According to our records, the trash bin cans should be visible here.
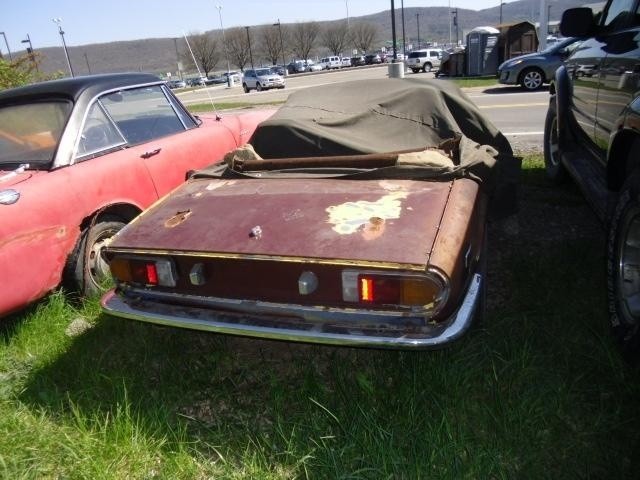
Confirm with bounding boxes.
[285,69,288,76]
[227,76,234,87]
